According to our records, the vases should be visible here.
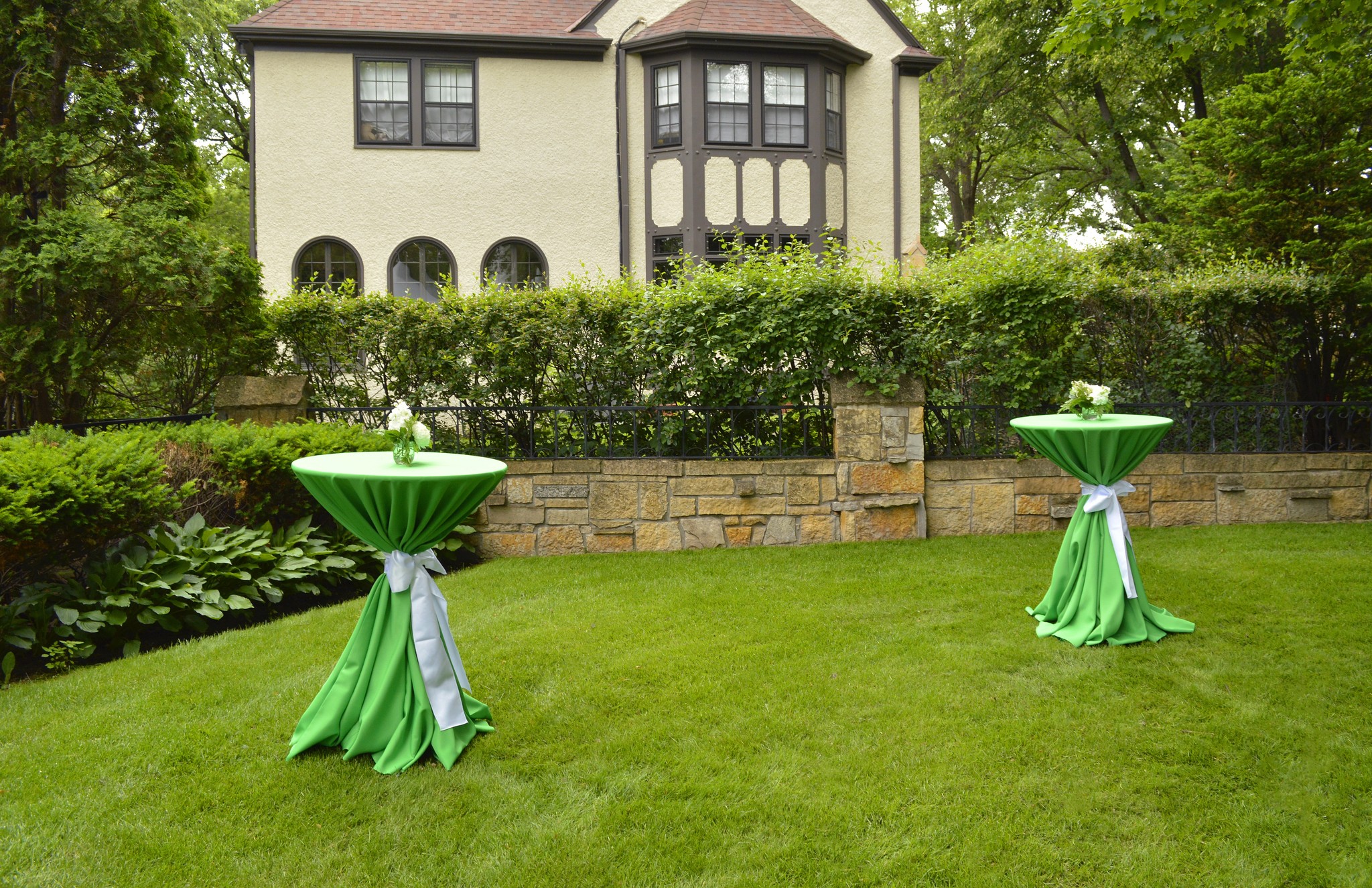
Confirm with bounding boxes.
[393,441,414,466]
[1082,406,1098,420]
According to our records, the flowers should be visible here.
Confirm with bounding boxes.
[384,400,433,451]
[1057,380,1114,419]
